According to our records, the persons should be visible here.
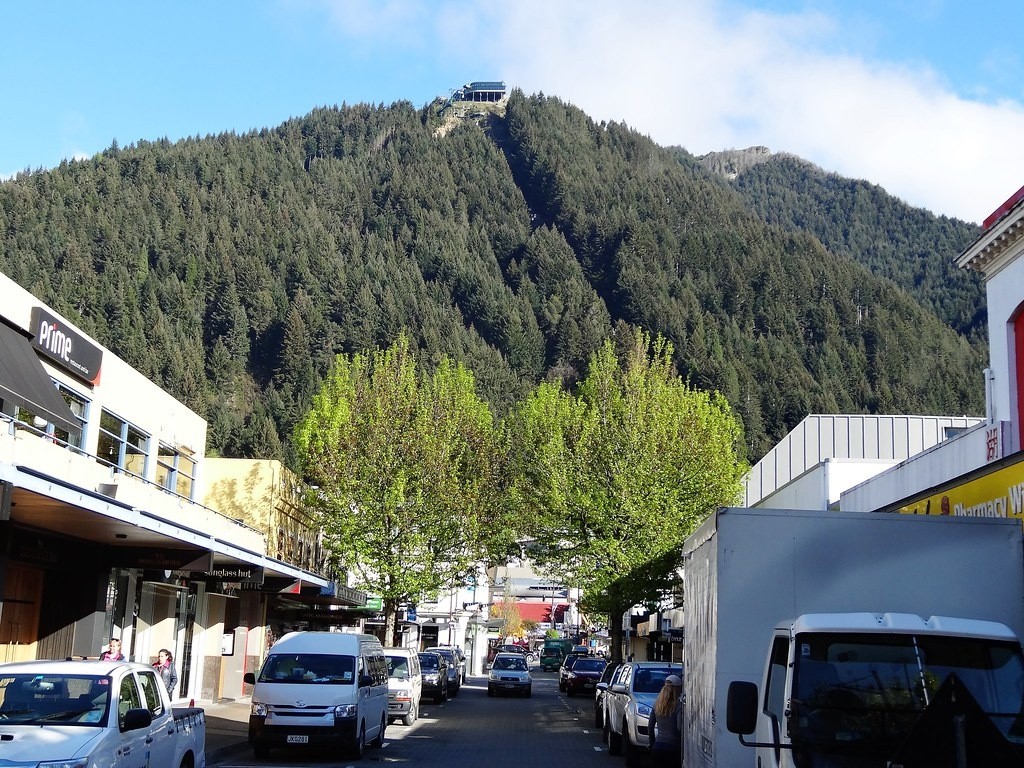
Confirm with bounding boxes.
[646,676,681,768]
[152,649,178,702]
[511,659,527,670]
[96,639,126,685]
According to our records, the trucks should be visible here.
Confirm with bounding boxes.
[540,639,572,672]
[681,505,1024,768]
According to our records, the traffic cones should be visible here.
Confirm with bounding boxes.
[189,699,194,708]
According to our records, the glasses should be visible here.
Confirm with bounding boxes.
[111,638,119,642]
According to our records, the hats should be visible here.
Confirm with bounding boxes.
[665,676,682,686]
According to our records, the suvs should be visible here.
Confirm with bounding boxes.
[486,653,533,697]
[565,657,609,697]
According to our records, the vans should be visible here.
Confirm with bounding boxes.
[244,632,389,764]
[383,647,422,726]
[571,645,588,657]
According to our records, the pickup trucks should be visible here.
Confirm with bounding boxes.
[0,660,206,768]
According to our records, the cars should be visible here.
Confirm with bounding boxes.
[559,654,589,692]
[418,652,448,704]
[423,647,463,697]
[497,644,536,662]
[607,661,684,760]
[594,663,621,729]
[596,664,624,744]
